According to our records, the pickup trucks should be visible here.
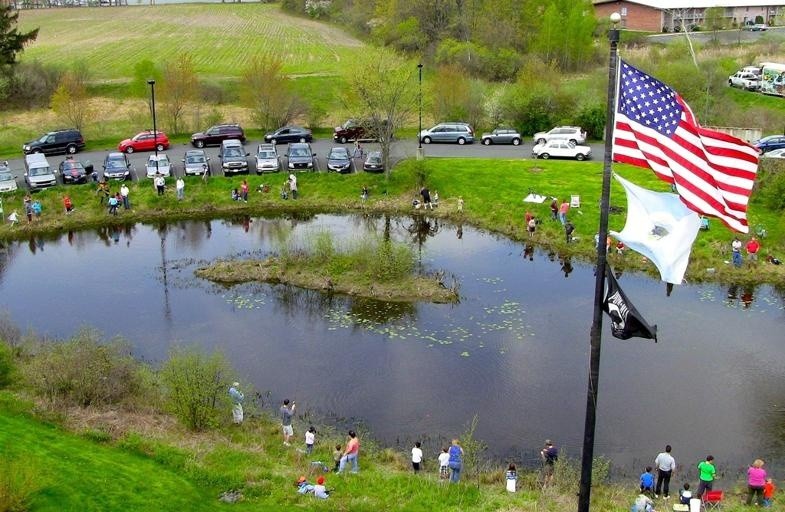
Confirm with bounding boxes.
[728,70,762,92]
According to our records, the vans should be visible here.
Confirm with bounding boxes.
[332,118,393,143]
[741,66,762,86]
[415,121,473,146]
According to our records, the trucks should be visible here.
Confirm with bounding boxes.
[758,62,785,97]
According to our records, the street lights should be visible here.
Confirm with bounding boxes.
[415,61,425,161]
[146,79,162,189]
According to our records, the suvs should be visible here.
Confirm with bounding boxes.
[23,152,58,192]
[102,152,131,182]
[325,147,353,173]
[254,141,281,174]
[748,23,767,31]
[22,126,86,156]
[0,160,18,196]
[283,143,317,172]
[191,123,246,148]
[529,122,587,145]
[218,138,249,175]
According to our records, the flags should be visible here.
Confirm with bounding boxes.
[611,171,703,286]
[599,261,660,343]
[611,55,762,233]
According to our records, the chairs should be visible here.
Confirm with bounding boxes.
[571,195,579,207]
[701,490,722,512]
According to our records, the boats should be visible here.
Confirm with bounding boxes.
[12,0,127,7]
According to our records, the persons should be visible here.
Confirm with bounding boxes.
[523,197,576,244]
[412,437,465,484]
[155,161,209,201]
[748,236,760,271]
[413,186,438,213]
[595,232,625,257]
[94,180,129,215]
[457,194,466,213]
[352,139,369,159]
[732,238,742,268]
[7,191,75,228]
[630,441,776,510]
[361,187,368,200]
[230,171,301,203]
[505,462,519,495]
[540,439,558,491]
[226,382,359,504]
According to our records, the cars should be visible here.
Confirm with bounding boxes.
[363,150,384,172]
[480,126,522,145]
[750,134,785,154]
[530,138,593,161]
[118,128,169,153]
[700,216,709,230]
[263,124,313,144]
[143,154,173,178]
[762,148,785,158]
[180,149,210,176]
[57,159,86,185]
